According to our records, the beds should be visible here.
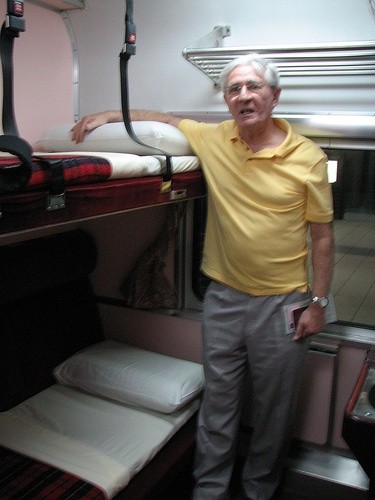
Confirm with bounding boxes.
[0,135,205,500]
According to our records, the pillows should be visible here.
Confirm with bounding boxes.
[52,340,205,414]
[34,121,194,156]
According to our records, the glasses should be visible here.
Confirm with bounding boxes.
[223,80,266,95]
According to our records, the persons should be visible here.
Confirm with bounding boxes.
[70,55,335,500]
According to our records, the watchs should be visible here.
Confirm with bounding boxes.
[311,297,329,308]
